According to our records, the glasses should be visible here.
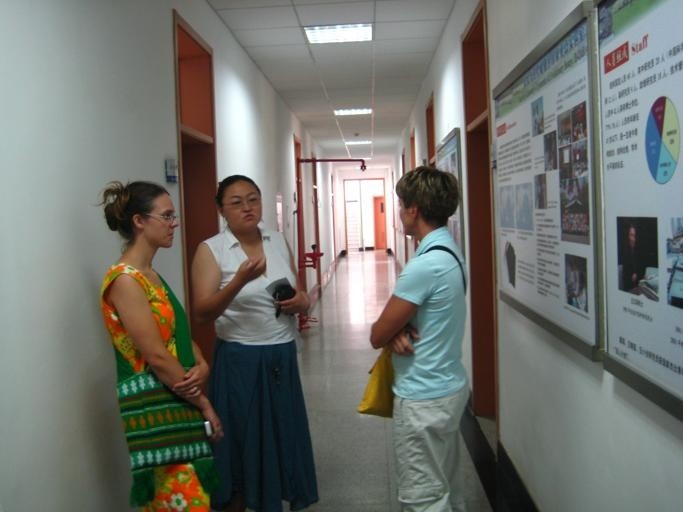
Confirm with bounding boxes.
[143,213,179,225]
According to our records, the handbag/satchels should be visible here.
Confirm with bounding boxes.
[358,342,395,416]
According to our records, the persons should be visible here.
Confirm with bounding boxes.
[189,173,318,511]
[97,179,228,512]
[367,164,471,512]
